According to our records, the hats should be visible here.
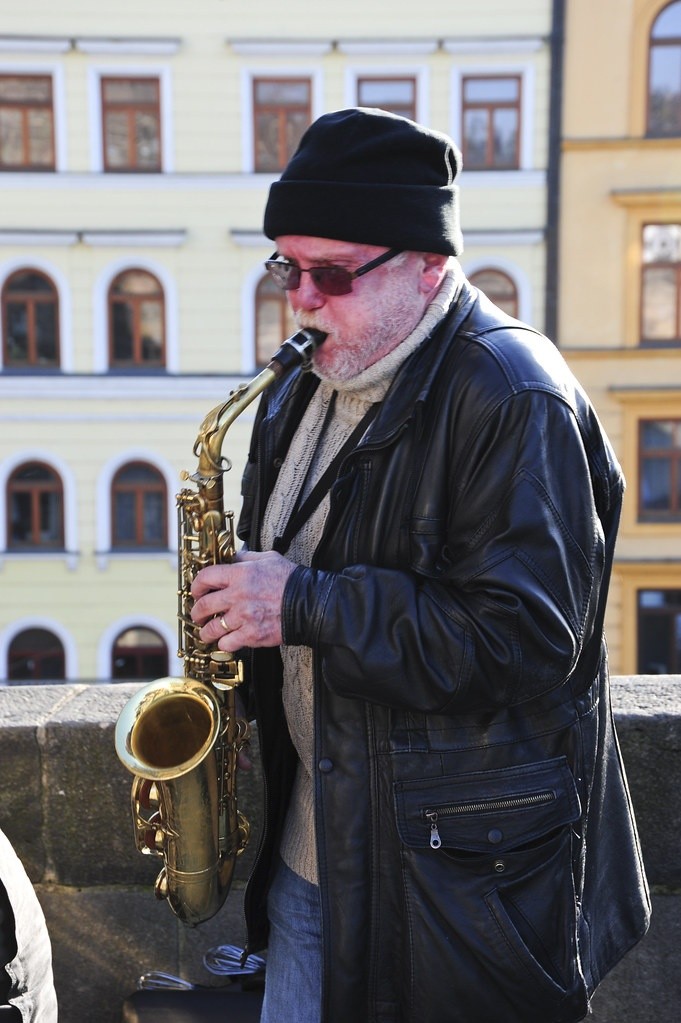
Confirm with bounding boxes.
[263,108,464,256]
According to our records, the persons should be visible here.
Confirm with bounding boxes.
[191,107,653,1023]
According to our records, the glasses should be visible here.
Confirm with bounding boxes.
[265,249,403,298]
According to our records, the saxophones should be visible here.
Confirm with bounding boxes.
[115,325,328,928]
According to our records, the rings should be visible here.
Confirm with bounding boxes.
[219,616,231,632]
